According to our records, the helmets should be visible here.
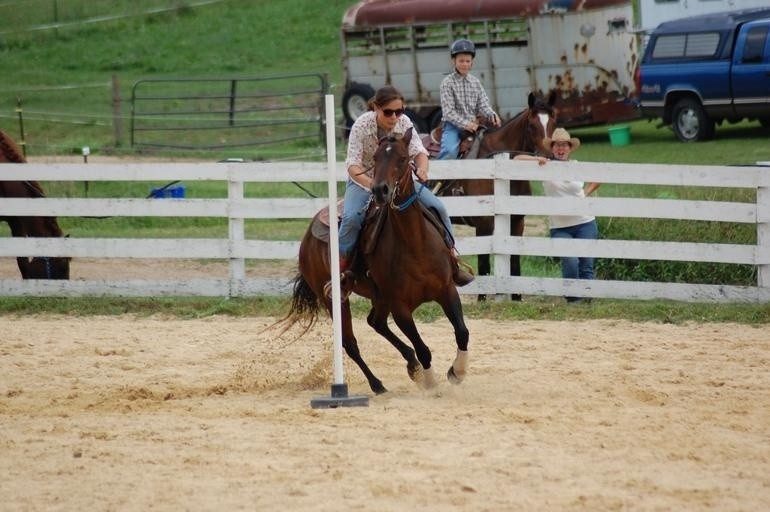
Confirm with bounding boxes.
[451,38,476,57]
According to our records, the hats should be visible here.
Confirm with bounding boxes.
[542,127,581,154]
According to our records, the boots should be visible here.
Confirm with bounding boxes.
[322,256,350,301]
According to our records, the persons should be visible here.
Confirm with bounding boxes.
[434,37,503,161]
[512,127,603,305]
[323,84,477,302]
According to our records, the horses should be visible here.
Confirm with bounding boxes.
[0,129,73,280]
[416,90,558,301]
[258,126,470,395]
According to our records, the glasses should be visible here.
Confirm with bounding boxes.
[377,104,405,117]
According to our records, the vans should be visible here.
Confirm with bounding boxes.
[635,9,770,142]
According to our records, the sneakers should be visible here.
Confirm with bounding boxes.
[449,250,475,288]
